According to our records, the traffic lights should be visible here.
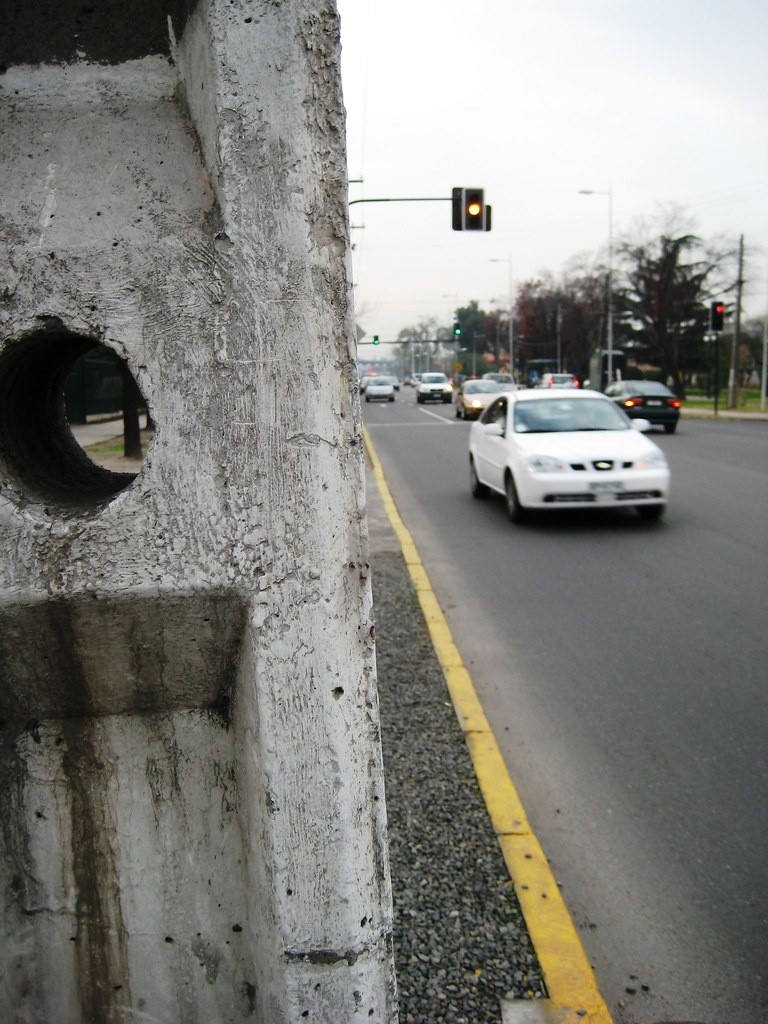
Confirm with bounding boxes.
[452,186,487,231]
[710,300,726,332]
[452,322,461,343]
[372,334,379,345]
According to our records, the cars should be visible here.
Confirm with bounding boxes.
[453,378,504,420]
[357,372,580,404]
[604,379,681,436]
[468,387,670,529]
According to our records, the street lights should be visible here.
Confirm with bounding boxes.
[490,258,514,378]
[576,181,615,385]
